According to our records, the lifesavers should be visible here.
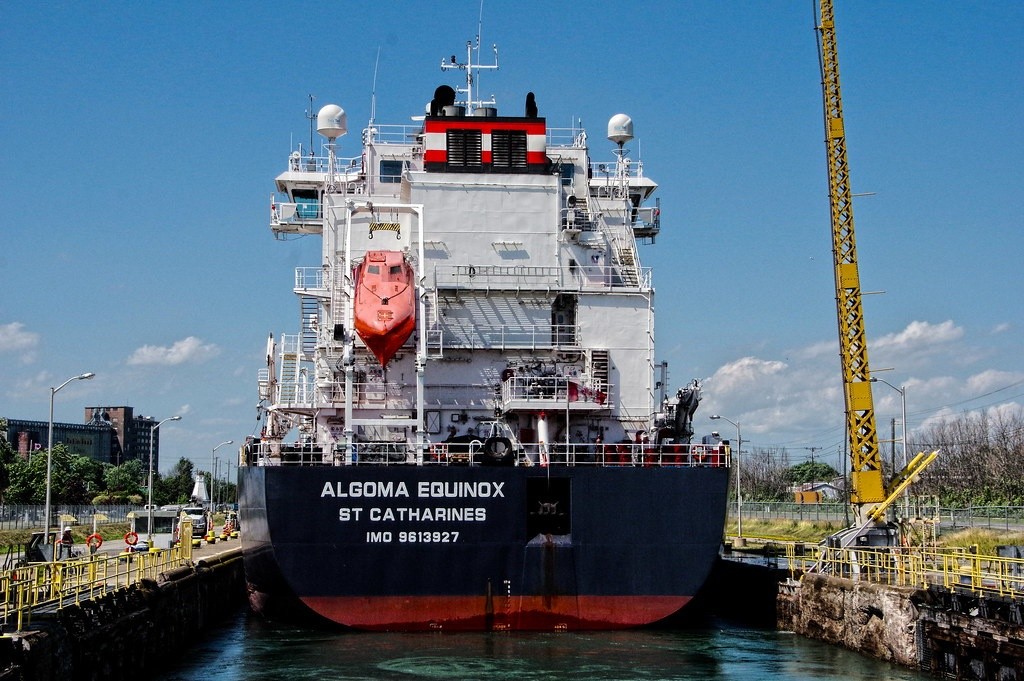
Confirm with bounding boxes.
[692,445,708,460]
[124,532,138,545]
[484,436,513,462]
[85,534,102,548]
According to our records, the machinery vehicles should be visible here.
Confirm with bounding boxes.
[813,0,942,574]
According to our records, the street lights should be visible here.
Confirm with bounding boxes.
[147,416,182,540]
[709,415,742,538]
[210,440,233,513]
[44,372,96,545]
[870,376,910,520]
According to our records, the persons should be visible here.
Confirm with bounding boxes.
[55,526,74,544]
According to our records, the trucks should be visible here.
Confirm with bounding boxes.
[182,507,207,538]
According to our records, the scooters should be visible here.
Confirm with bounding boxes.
[15,531,86,575]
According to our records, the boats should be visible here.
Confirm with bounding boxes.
[354,250,417,369]
[235,0,733,634]
